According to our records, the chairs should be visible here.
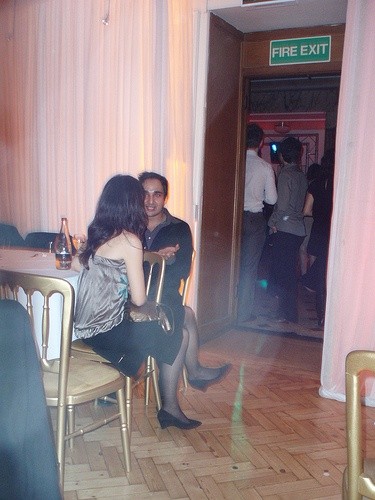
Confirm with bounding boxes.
[0,224,196,500]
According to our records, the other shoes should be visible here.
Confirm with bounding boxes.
[239,313,256,323]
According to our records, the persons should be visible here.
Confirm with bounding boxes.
[99,172,194,404]
[239,123,277,321]
[73,174,231,430]
[264,137,328,323]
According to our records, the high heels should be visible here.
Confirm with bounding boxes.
[157,408,199,430]
[190,363,228,392]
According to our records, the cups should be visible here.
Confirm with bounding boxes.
[73,233,86,252]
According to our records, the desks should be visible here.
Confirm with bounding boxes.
[1,248,82,359]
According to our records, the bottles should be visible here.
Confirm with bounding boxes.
[55,217,72,270]
